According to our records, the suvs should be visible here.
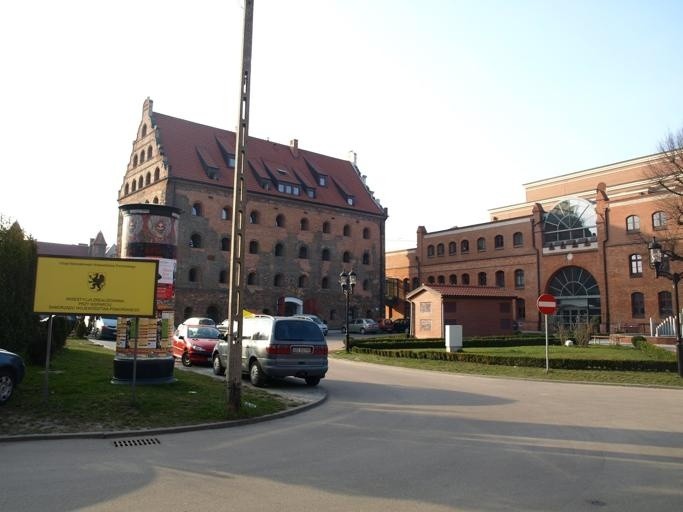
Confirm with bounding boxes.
[211,314,327,387]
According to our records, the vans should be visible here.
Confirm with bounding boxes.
[290,314,327,336]
[177,318,217,333]
[82,316,117,340]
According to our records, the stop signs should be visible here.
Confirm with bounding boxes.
[536,293,556,315]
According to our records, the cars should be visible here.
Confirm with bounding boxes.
[340,316,409,334]
[172,324,222,367]
[215,319,237,338]
[0,348,25,405]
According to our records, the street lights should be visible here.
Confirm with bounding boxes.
[647,235,683,377]
[339,267,356,354]
